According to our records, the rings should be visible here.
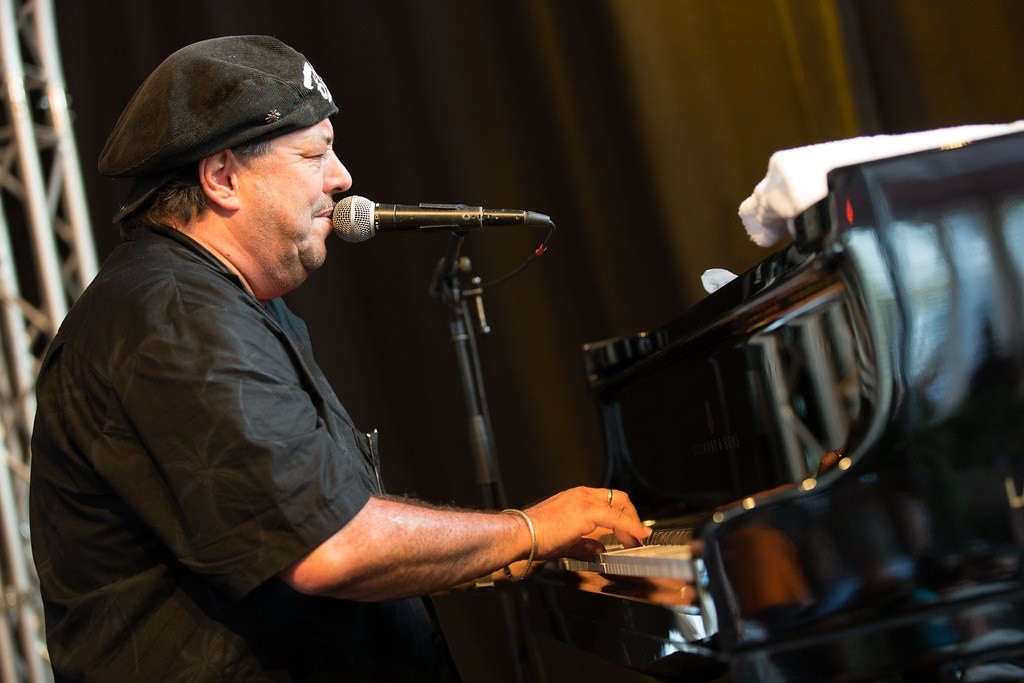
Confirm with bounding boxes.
[608,488,614,505]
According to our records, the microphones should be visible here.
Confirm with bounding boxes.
[333,196,552,244]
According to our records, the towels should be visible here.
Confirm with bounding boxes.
[738,120,1024,248]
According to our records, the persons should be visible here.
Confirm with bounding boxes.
[28,32,658,683]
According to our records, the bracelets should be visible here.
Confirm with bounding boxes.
[499,509,539,584]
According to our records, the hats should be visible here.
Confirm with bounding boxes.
[95,35,339,181]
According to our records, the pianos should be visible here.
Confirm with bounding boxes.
[550,128,1024,683]
